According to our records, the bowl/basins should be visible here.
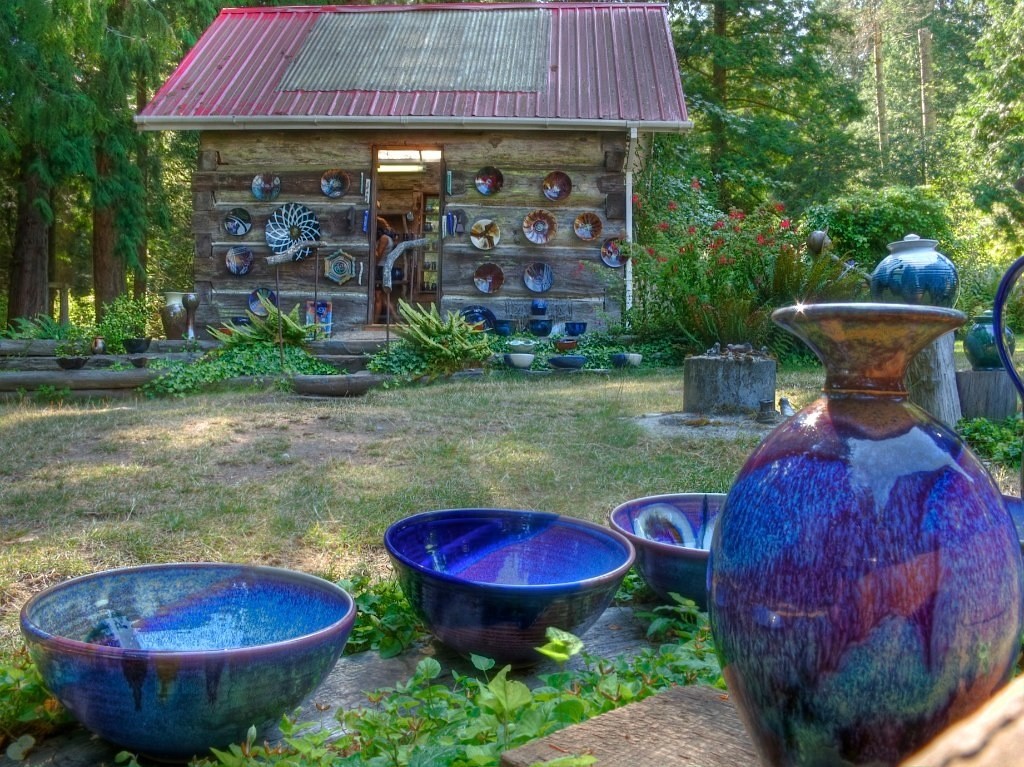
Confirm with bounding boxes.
[608,354,643,369]
[1002,494,1024,555]
[384,508,636,670]
[391,267,402,280]
[506,340,539,353]
[609,493,729,612]
[218,316,250,335]
[503,354,535,369]
[121,337,160,368]
[553,338,577,351]
[493,319,518,337]
[564,322,587,336]
[55,357,89,370]
[531,300,548,315]
[547,356,587,369]
[530,319,553,337]
[19,562,357,760]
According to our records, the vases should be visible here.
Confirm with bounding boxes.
[708,305,1024,767]
[160,291,198,340]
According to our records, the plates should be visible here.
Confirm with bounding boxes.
[542,171,572,201]
[475,166,503,195]
[458,305,496,331]
[473,262,505,294]
[248,288,277,317]
[305,301,333,340]
[573,212,632,267]
[224,169,350,276]
[522,209,559,245]
[470,219,500,250]
[523,263,554,292]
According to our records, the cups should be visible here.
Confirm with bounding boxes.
[424,262,436,270]
[425,222,431,232]
[421,282,436,291]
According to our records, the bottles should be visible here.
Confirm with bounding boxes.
[963,309,1016,371]
[706,303,1024,767]
[91,336,106,355]
[870,233,961,308]
[160,292,188,340]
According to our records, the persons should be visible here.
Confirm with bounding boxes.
[375,216,400,323]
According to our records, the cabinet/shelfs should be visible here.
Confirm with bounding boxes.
[417,212,438,294]
